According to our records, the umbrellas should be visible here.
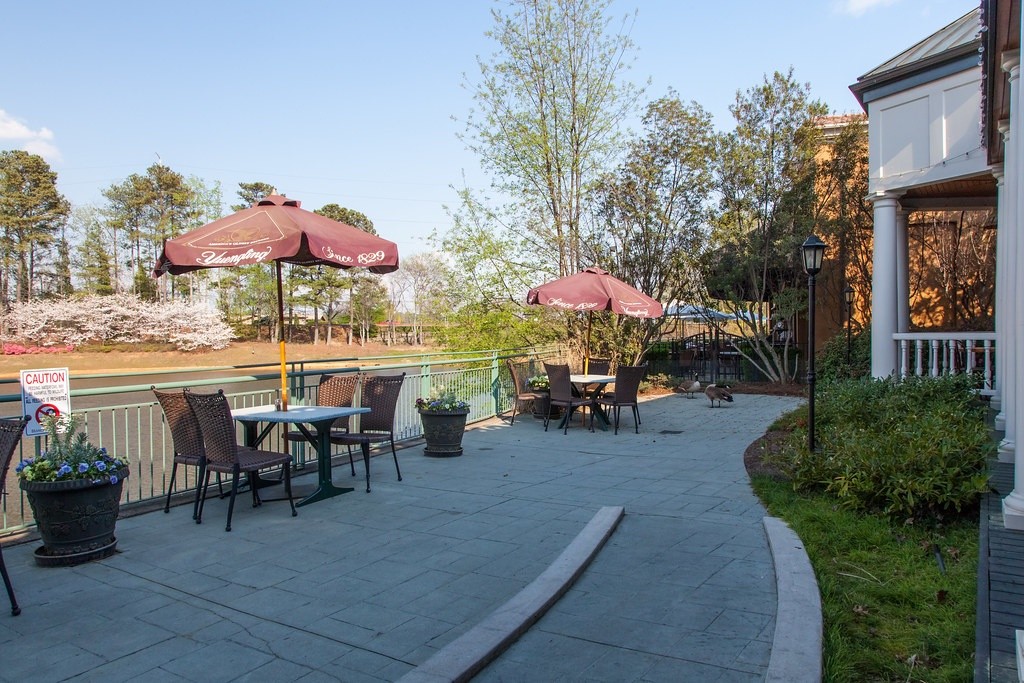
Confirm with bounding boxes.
[661,299,764,338]
[526,267,663,426]
[152,194,399,494]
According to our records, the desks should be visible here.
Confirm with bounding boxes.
[669,340,752,382]
[223,404,372,509]
[544,374,617,431]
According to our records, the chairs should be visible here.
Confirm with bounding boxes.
[279,372,407,493]
[672,338,726,381]
[151,385,298,532]
[0,414,32,616]
[507,355,648,435]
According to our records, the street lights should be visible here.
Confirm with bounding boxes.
[844,281,855,379]
[803,234,829,452]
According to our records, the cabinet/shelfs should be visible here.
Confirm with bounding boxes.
[20,466,130,555]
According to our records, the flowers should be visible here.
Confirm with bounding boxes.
[15,414,131,485]
[527,375,550,390]
[412,391,470,412]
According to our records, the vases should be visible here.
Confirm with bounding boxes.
[418,408,470,457]
[528,386,560,415]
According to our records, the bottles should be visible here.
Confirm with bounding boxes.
[275,399,282,411]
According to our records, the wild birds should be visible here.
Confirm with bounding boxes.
[677,371,700,399]
[704,384,734,408]
